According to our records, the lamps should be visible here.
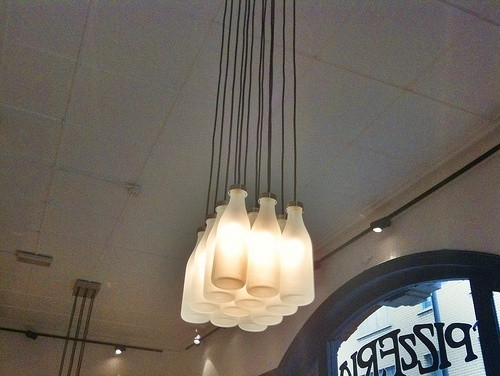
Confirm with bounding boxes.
[181,2,318,335]
